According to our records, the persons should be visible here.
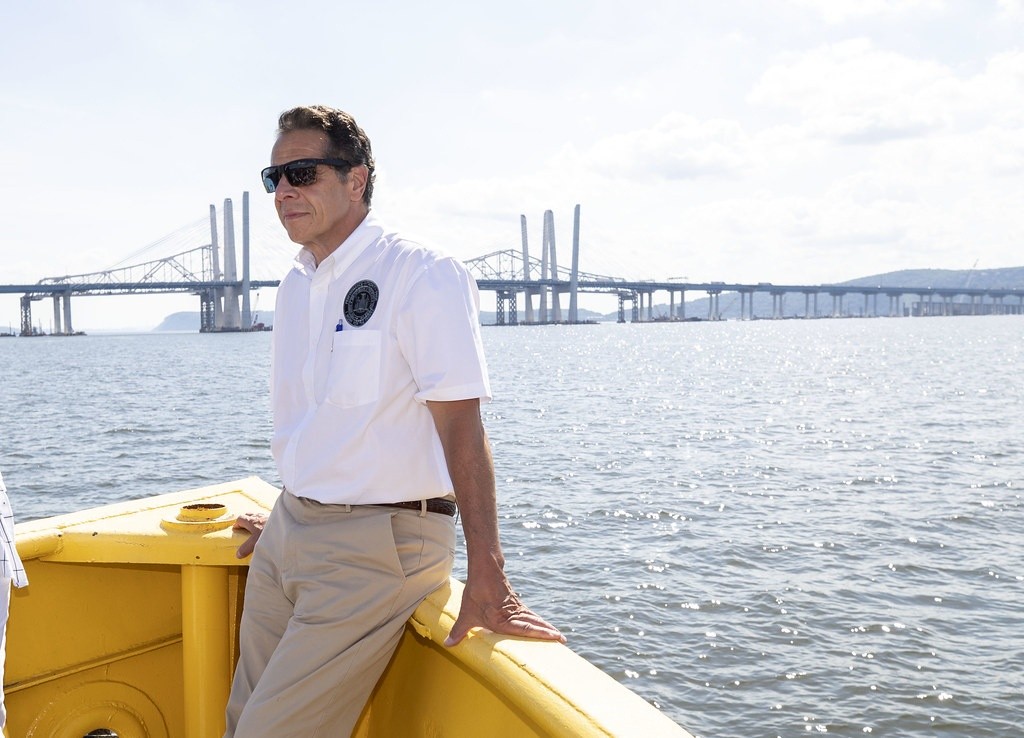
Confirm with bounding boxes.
[0,471,29,738]
[222,104,567,738]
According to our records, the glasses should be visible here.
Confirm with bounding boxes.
[261,158,351,194]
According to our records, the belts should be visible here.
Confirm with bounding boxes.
[369,497,456,517]
